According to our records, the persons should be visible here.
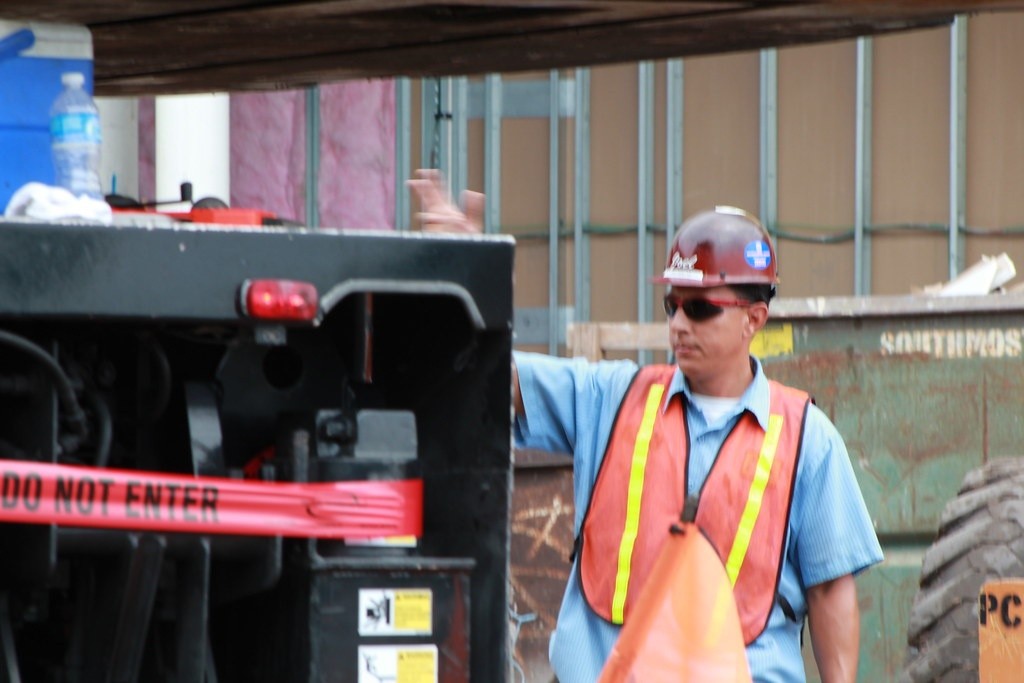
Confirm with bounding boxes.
[408,167,884,683]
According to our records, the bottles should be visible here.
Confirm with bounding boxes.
[50,71,102,202]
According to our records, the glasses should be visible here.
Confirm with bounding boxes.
[662,295,751,323]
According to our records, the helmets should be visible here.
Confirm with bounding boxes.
[649,205,779,288]
